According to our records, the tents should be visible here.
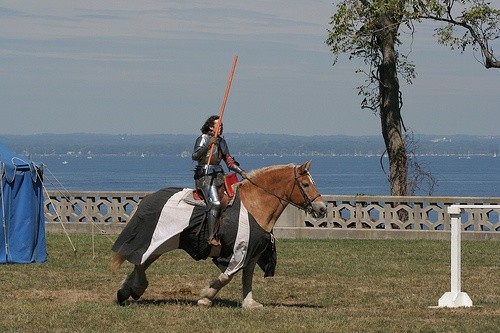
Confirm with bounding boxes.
[0,143,116,264]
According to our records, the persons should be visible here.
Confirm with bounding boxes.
[192,115,246,246]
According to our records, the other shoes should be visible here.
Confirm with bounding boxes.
[208,240,221,247]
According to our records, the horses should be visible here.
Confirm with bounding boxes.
[110,160,328,310]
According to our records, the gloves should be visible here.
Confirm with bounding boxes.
[206,136,218,150]
[241,171,248,179]
